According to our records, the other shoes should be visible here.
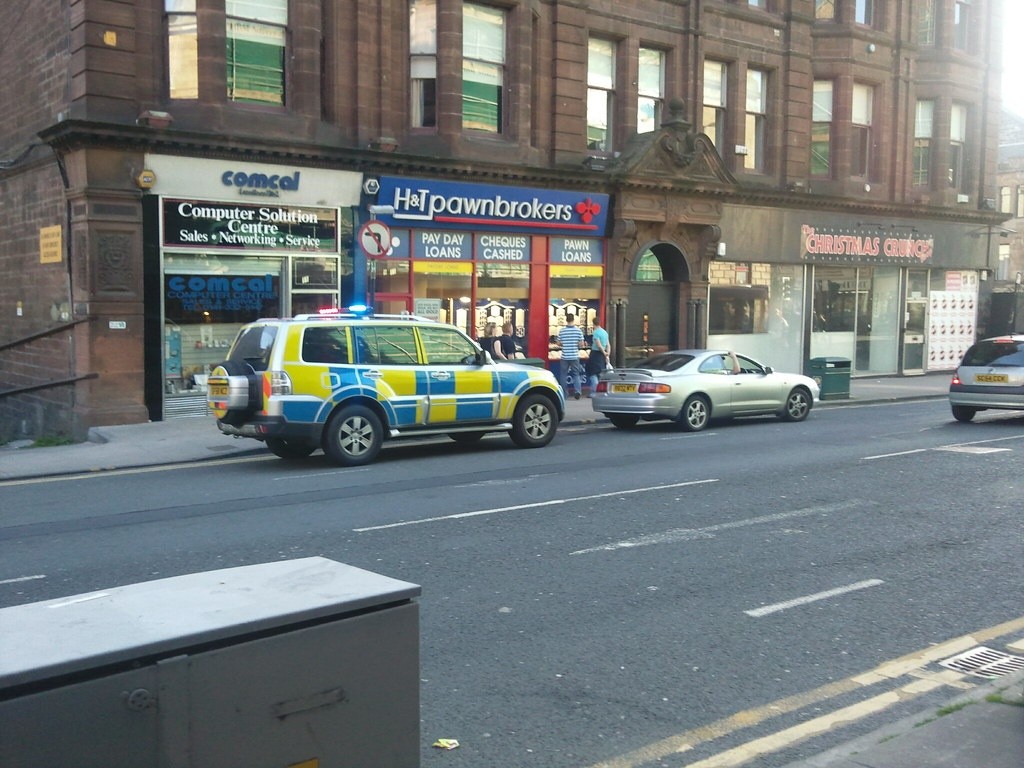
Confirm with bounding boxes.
[575,392,579,399]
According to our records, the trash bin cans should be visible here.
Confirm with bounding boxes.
[809,357,852,401]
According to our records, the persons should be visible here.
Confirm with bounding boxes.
[721,349,741,375]
[586,318,611,399]
[767,306,790,333]
[476,322,516,364]
[556,313,585,400]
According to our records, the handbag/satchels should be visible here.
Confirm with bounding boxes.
[606,363,614,373]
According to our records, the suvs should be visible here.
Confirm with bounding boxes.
[205,305,566,467]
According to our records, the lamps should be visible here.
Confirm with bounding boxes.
[370,136,400,152]
[138,110,177,128]
[786,182,808,193]
[858,221,886,231]
[976,230,1010,238]
[891,224,919,233]
[915,194,931,205]
[583,155,611,171]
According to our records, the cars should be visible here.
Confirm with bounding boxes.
[949,334,1024,422]
[592,348,821,433]
